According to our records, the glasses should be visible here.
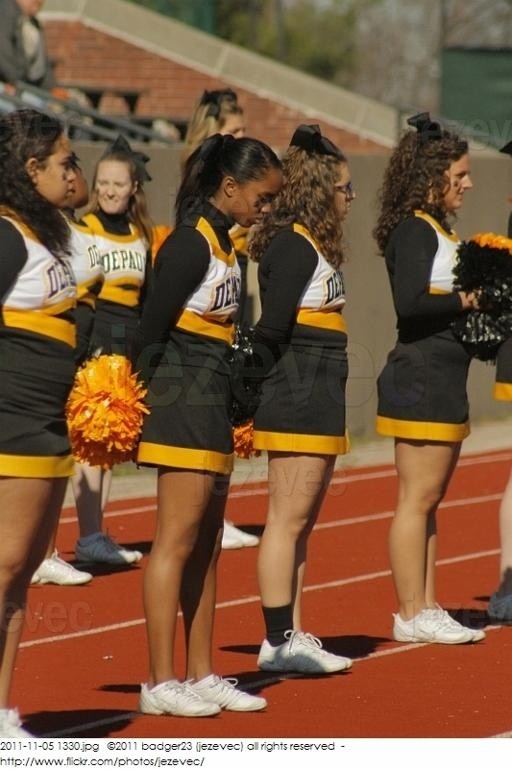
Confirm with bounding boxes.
[333,180,353,201]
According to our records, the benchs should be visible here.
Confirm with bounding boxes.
[54,80,149,115]
[60,109,190,145]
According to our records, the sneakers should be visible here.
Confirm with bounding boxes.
[487,591,512,621]
[30,548,94,586]
[391,609,474,645]
[0,705,36,738]
[185,673,267,712]
[432,601,486,642]
[221,534,243,550]
[297,631,353,669]
[256,629,347,674]
[139,677,222,718]
[74,526,137,565]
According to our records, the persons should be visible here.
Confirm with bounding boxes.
[0,0,48,96]
[487,139,511,622]
[373,112,512,645]
[230,123,357,678]
[179,88,250,325]
[74,134,156,565]
[30,151,105,586]
[65,133,283,719]
[0,108,77,739]
